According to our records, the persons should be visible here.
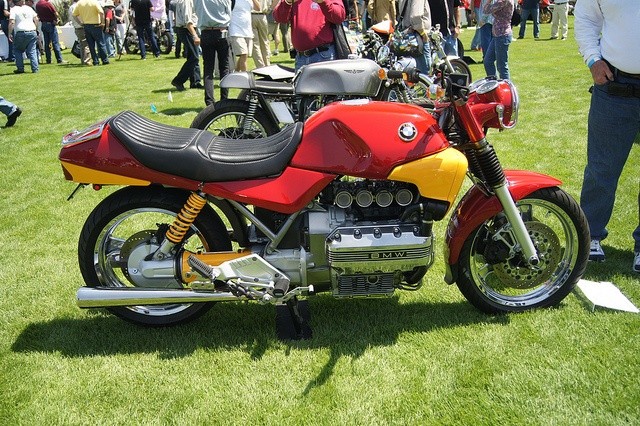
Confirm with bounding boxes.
[479,1,494,64]
[0,97,21,130]
[250,0,273,75]
[445,0,464,61]
[432,0,451,53]
[0,0,12,59]
[36,0,63,65]
[548,0,569,41]
[173,0,204,91]
[193,0,230,106]
[226,0,261,73]
[571,0,640,274]
[399,0,433,75]
[6,0,39,74]
[482,0,515,87]
[347,0,398,36]
[67,0,170,65]
[270,0,345,75]
[516,0,542,41]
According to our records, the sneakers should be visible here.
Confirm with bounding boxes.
[587,239,607,263]
[632,249,640,271]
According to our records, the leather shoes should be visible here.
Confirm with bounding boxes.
[5,107,21,126]
[171,80,186,91]
[190,83,204,88]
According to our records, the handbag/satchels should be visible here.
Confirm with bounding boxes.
[332,23,352,59]
[511,3,523,26]
[390,0,424,57]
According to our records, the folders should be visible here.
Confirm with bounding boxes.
[574,278,640,315]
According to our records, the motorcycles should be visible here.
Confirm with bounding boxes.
[432,25,472,88]
[58,69,591,324]
[184,31,473,140]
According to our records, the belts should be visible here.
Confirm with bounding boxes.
[251,12,266,15]
[43,22,51,24]
[296,46,328,57]
[204,27,226,30]
[554,1,567,4]
[605,62,638,79]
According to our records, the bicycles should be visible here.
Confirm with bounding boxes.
[123,17,172,55]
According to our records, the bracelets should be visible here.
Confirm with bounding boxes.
[587,56,604,68]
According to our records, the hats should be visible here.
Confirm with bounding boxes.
[102,0,115,7]
